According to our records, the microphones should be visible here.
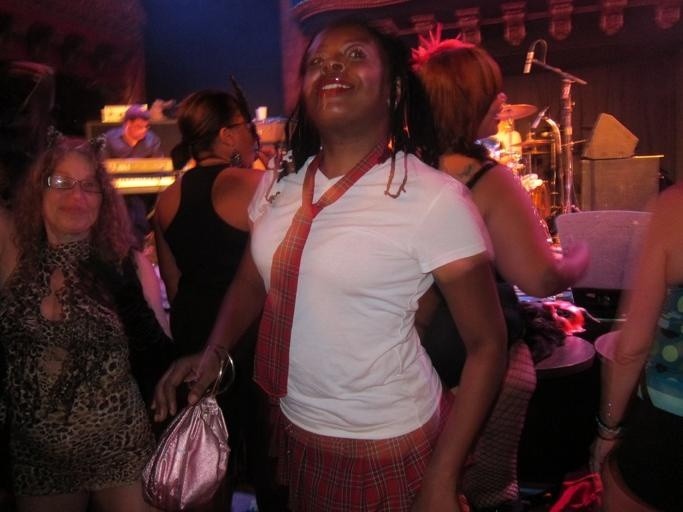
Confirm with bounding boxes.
[523,49,534,75]
[532,106,551,130]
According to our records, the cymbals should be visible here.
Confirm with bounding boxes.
[498,102,539,122]
[520,150,548,156]
[511,138,553,147]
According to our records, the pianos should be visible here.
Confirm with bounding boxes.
[102,156,198,195]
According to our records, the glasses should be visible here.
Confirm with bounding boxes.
[45,173,104,194]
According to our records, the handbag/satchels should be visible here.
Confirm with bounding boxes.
[140,393,232,511]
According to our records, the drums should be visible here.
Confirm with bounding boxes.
[519,174,552,219]
